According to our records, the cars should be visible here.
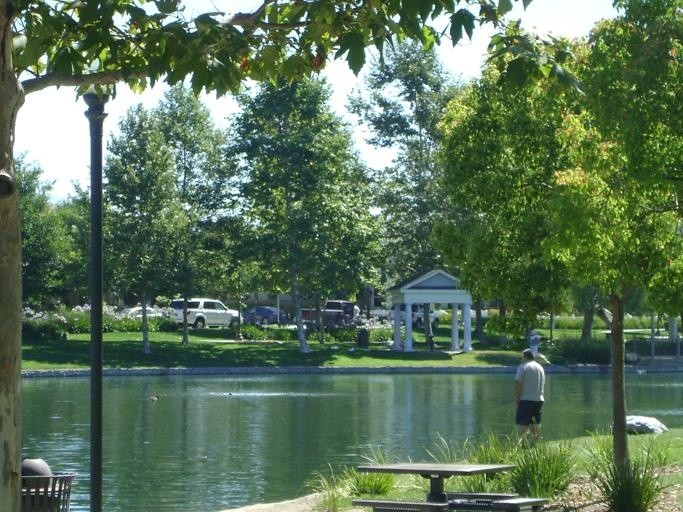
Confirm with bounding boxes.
[242,305,290,327]
[120,306,163,318]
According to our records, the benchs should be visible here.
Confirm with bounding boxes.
[352,500,449,512]
[426,493,519,512]
[492,498,549,512]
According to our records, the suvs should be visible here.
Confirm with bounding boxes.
[387,303,439,330]
[169,298,243,329]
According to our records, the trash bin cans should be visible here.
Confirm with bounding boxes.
[357,329,369,348]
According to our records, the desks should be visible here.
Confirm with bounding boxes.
[357,463,517,502]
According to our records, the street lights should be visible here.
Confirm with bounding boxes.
[78,62,114,512]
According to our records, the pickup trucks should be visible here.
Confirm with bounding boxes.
[301,299,356,329]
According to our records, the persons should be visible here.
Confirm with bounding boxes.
[513,347,546,449]
[528,330,541,355]
